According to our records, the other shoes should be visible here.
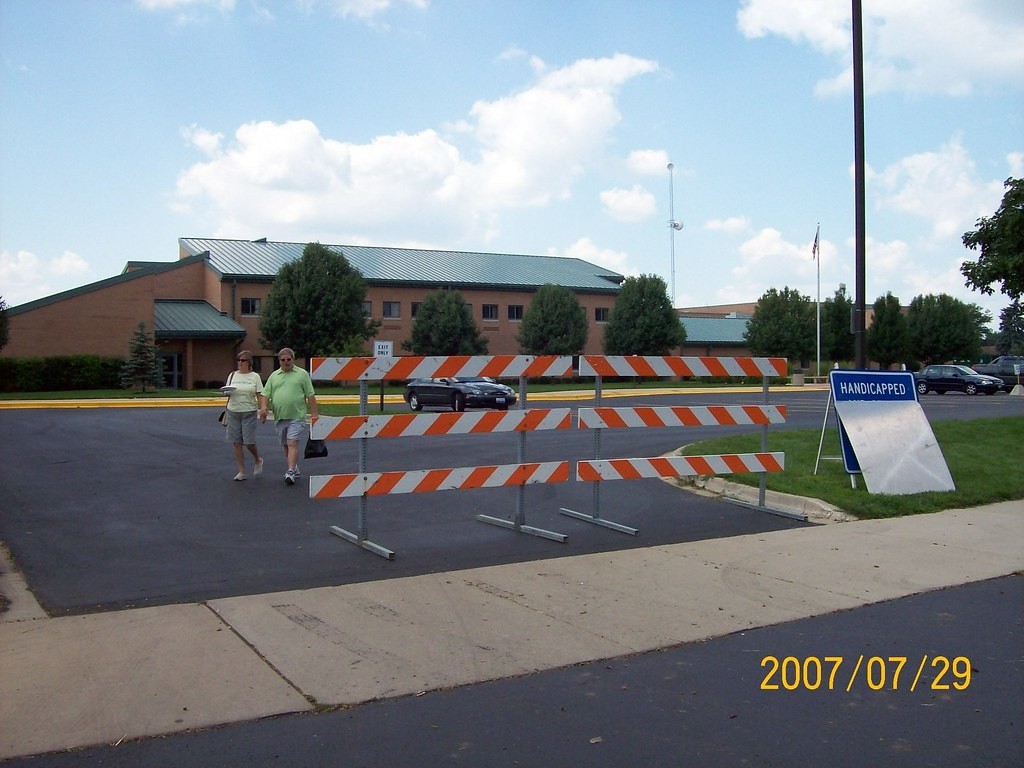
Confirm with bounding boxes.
[284,469,295,485]
[234,472,247,481]
[295,468,300,477]
[253,458,263,475]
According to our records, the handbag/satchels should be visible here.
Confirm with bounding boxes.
[304,440,328,459]
[219,411,227,426]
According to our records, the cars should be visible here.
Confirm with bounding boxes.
[970,356,1024,392]
[912,365,1005,395]
[402,377,517,412]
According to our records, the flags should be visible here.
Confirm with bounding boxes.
[812,229,818,261]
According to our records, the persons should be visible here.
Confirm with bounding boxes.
[833,361,839,369]
[224,350,266,481]
[260,348,318,485]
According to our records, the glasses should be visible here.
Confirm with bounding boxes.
[237,359,247,362]
[281,358,292,361]
